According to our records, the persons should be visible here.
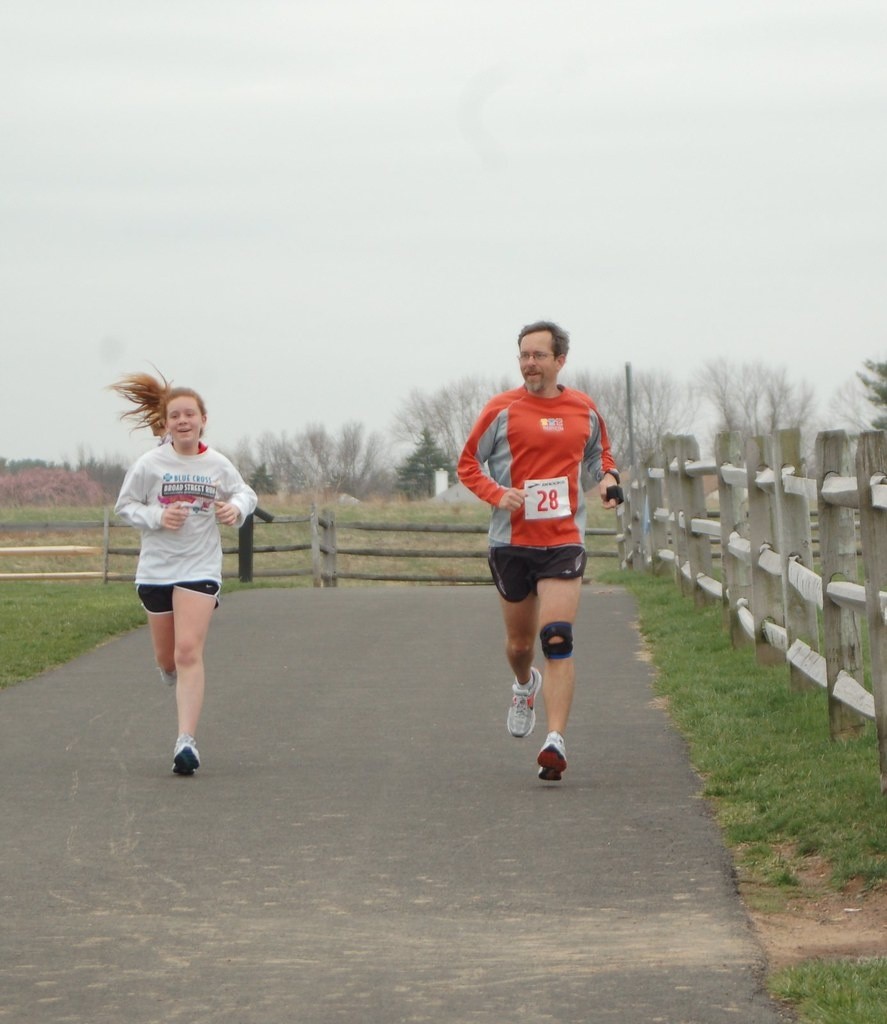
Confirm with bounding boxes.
[458,321,624,781]
[110,359,257,775]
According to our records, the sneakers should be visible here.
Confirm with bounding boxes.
[536,730,567,780]
[171,731,200,776]
[159,666,177,686]
[507,666,543,738]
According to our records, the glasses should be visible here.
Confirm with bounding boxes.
[516,351,554,361]
